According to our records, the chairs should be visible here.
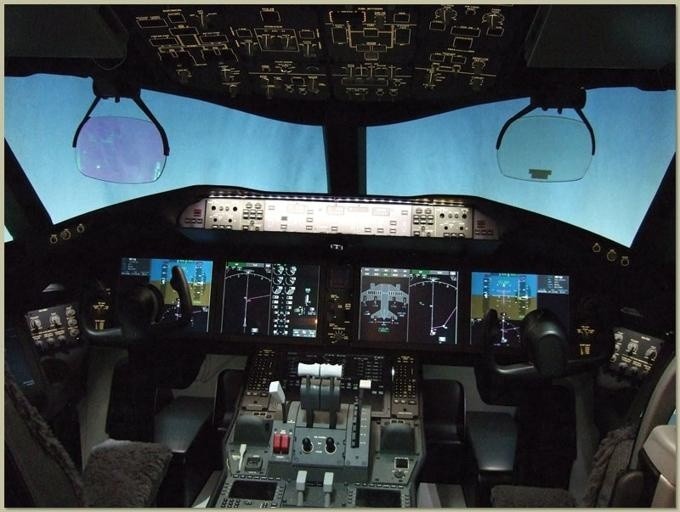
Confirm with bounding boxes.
[5,349,175,508]
[488,333,675,507]
